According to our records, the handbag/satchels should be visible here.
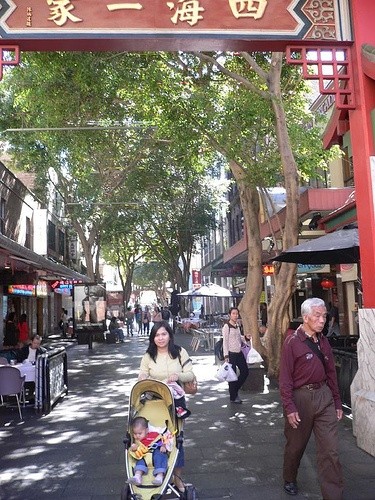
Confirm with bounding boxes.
[215,363,238,382]
[184,371,197,394]
[246,338,264,364]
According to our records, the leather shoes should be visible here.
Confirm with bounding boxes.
[283,481,297,495]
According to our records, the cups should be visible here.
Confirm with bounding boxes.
[10,358,30,365]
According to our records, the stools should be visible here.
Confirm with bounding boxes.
[106,333,116,344]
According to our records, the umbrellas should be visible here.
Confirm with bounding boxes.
[176,282,243,314]
[269,224,362,309]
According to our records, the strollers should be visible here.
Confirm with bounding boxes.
[120,378,200,500]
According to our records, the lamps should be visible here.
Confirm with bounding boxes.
[308,212,322,230]
[266,240,274,255]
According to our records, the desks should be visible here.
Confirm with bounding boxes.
[0,364,35,408]
[202,328,220,349]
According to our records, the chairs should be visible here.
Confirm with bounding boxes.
[0,366,27,421]
[188,328,211,353]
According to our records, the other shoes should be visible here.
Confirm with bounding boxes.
[152,475,162,484]
[128,476,142,485]
[231,395,242,403]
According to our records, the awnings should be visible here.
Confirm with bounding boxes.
[0,232,91,283]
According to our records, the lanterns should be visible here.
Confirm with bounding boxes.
[221,265,243,276]
[321,278,335,290]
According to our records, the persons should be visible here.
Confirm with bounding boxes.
[222,307,251,403]
[58,308,69,337]
[109,316,124,343]
[135,305,170,336]
[138,321,193,493]
[201,305,204,315]
[125,417,168,486]
[260,303,268,327]
[327,301,340,336]
[279,298,343,500]
[4,312,30,346]
[16,334,47,404]
[125,307,134,336]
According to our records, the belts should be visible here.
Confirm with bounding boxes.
[298,380,326,392]
